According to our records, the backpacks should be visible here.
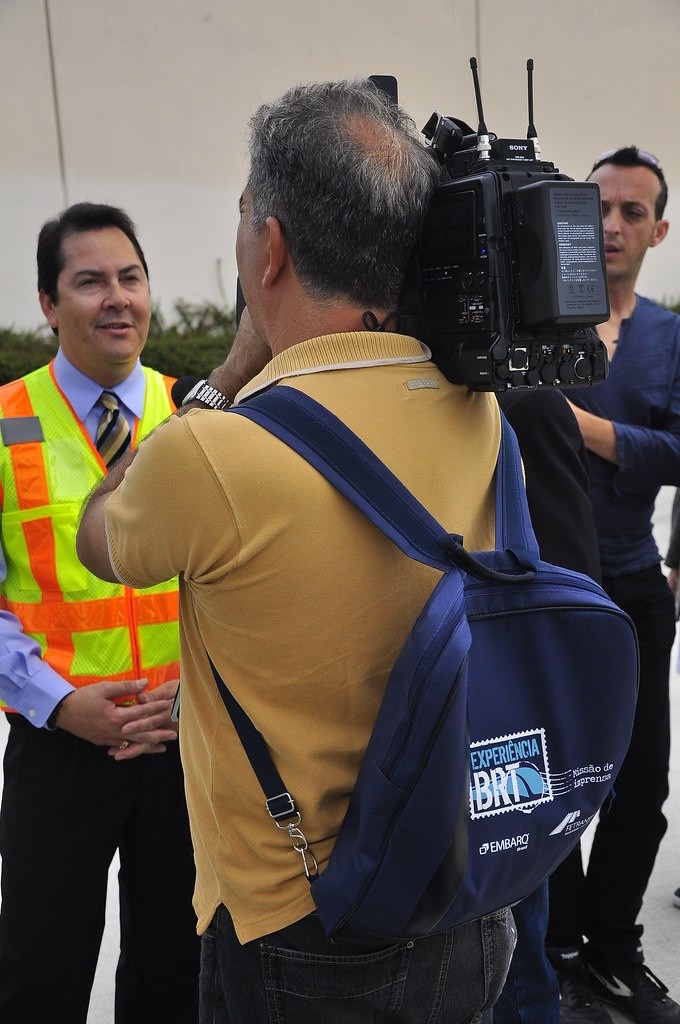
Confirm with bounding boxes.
[230,383,641,949]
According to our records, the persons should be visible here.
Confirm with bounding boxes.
[72,79,680,1024]
[0,200,203,1024]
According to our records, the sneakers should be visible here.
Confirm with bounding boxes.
[593,925,680,1024]
[546,943,614,1024]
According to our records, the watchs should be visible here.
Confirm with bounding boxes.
[182,379,231,411]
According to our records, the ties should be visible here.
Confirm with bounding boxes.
[94,390,132,475]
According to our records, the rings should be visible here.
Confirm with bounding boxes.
[120,741,130,749]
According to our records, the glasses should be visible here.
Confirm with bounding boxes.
[596,148,662,173]
